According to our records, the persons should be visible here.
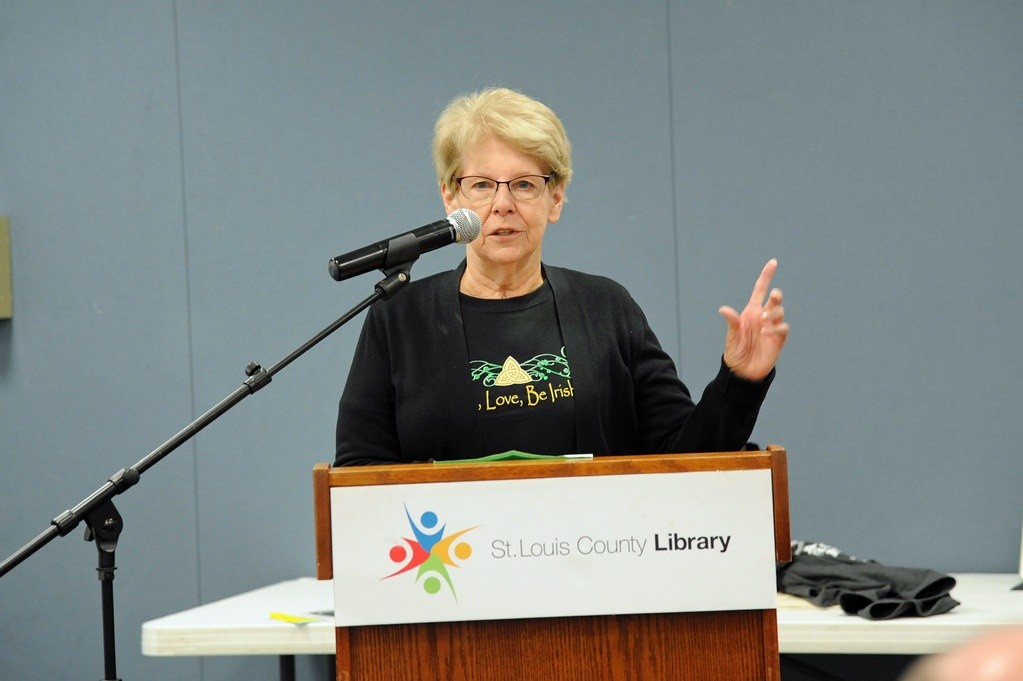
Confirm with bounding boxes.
[335,83,791,472]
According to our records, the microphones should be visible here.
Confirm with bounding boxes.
[327,207,482,281]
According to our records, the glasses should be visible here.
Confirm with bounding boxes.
[452,172,556,202]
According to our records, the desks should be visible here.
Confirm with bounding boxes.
[140,572,1023,681]
